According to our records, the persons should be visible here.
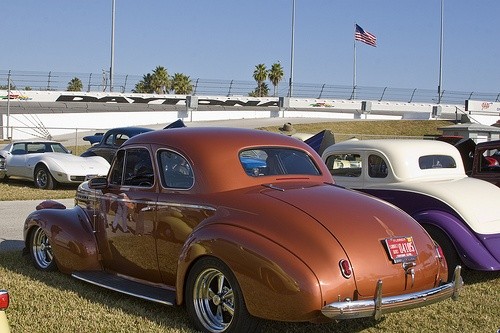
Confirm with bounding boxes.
[278,122,303,141]
[99,193,130,233]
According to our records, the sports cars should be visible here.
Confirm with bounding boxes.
[317,138,500,275]
[0,138,110,189]
[21,127,464,333]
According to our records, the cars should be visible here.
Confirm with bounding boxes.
[80,126,156,163]
[466,140,500,187]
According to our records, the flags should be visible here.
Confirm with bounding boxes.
[355,24,376,48]
